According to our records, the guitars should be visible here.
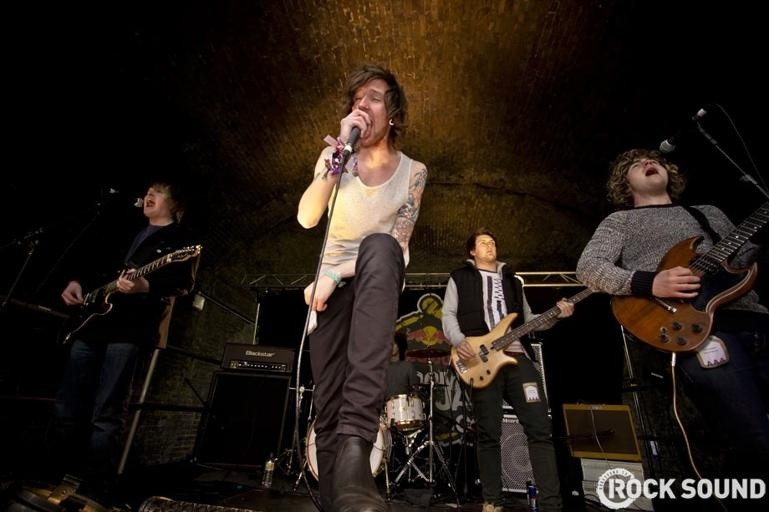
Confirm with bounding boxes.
[611,201,769,354]
[451,286,603,389]
[57,245,203,360]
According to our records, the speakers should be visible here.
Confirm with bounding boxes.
[191,370,294,477]
[501,344,548,410]
[496,414,553,495]
[562,403,642,462]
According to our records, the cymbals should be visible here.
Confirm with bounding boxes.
[414,384,450,388]
[407,348,452,359]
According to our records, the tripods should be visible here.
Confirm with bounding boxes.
[386,360,476,509]
[274,385,305,478]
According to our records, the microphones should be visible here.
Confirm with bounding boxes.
[659,105,707,153]
[110,187,144,207]
[342,127,361,156]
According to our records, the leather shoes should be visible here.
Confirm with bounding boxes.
[334,437,388,510]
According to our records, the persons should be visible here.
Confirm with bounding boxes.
[41,181,202,468]
[575,149,769,511]
[298,65,428,512]
[442,229,574,512]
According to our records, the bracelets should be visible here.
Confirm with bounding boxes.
[320,269,342,285]
[318,134,347,180]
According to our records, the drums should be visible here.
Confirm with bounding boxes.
[306,414,409,498]
[384,394,428,432]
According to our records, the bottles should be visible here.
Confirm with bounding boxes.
[262,452,276,488]
[525,480,534,507]
